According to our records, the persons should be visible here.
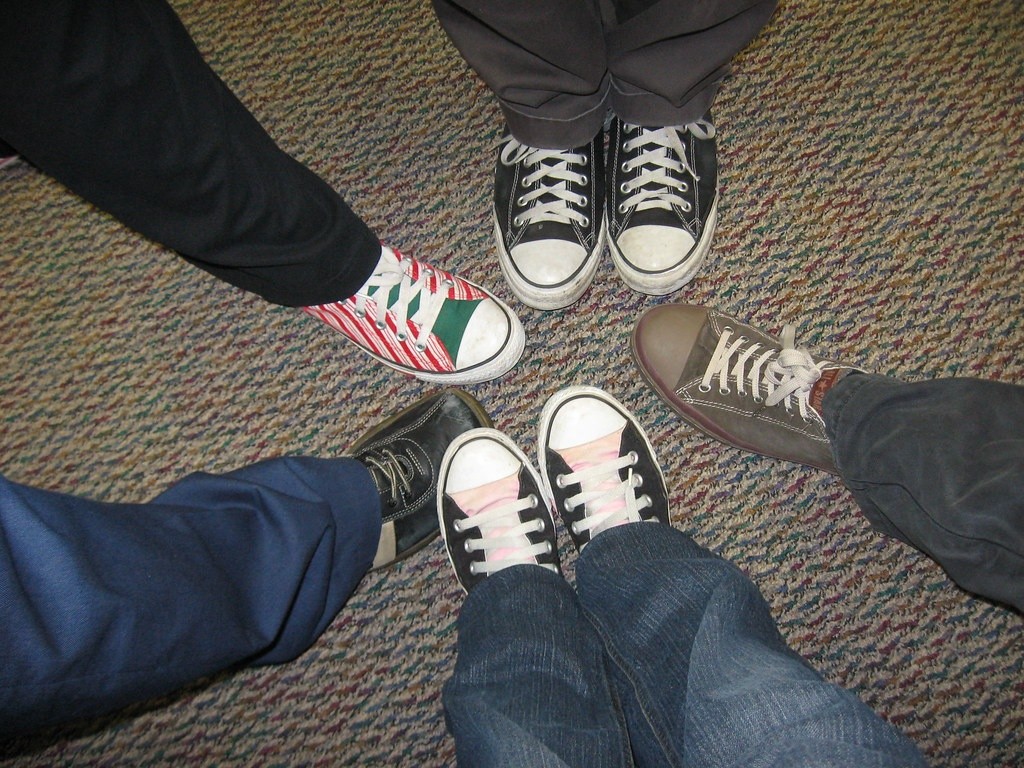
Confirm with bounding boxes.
[0,0,525,386]
[0,387,495,768]
[432,0,779,310]
[436,385,928,768]
[631,303,1024,611]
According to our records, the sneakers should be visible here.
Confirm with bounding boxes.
[630,302,869,476]
[537,384,672,554]
[339,389,492,574]
[486,122,609,311]
[608,108,719,295]
[302,241,526,386]
[438,428,564,597]
